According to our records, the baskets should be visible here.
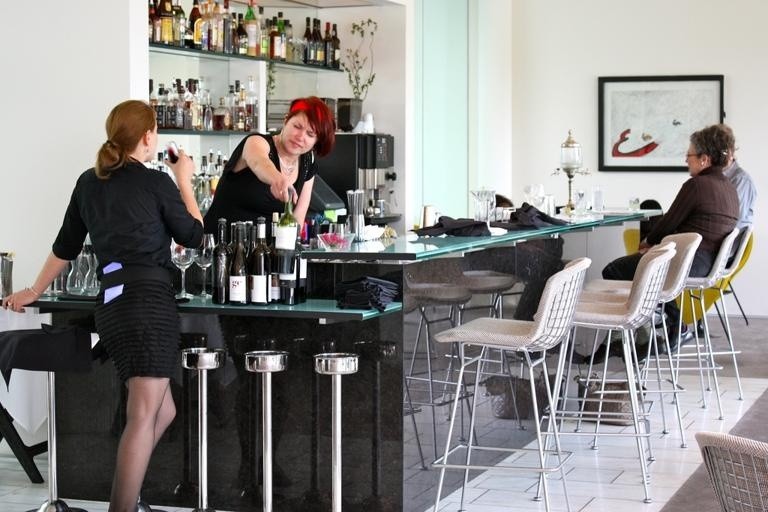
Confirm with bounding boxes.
[484,364,564,422]
[574,373,648,426]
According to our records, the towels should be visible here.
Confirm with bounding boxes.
[408,214,491,240]
[491,201,570,230]
[334,271,401,314]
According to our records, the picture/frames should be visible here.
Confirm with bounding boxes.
[597,74,725,172]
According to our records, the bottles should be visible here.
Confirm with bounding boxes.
[269,16,281,61]
[260,4,268,25]
[229,221,249,305]
[212,218,230,305]
[312,18,321,63]
[201,3,212,52]
[324,22,334,68]
[275,189,297,274]
[211,0,226,50]
[277,11,287,60]
[236,13,248,53]
[285,19,295,62]
[249,217,272,305]
[173,0,184,48]
[153,1,162,46]
[223,1,236,55]
[261,26,268,58]
[318,20,324,64]
[245,0,258,57]
[280,279,294,306]
[246,219,252,253]
[231,222,236,267]
[304,17,311,63]
[231,11,240,53]
[189,1,203,49]
[295,236,308,303]
[331,23,340,68]
[272,212,281,305]
[159,1,174,48]
[151,76,260,132]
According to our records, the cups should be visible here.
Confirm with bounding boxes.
[524,183,544,211]
[170,238,197,300]
[165,141,181,164]
[372,199,392,217]
[591,184,604,210]
[191,233,216,301]
[469,185,497,230]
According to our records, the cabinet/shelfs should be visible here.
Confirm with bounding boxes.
[148,1,405,163]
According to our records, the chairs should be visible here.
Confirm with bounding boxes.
[531,248,677,504]
[430,257,592,511]
[667,223,754,400]
[403,255,479,460]
[633,227,740,421]
[403,272,430,470]
[623,229,754,346]
[583,233,703,454]
[428,254,516,421]
[696,433,768,512]
[574,240,678,476]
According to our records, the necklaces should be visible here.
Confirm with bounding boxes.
[273,135,300,176]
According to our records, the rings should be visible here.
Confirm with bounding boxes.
[6,301,12,306]
[280,190,283,195]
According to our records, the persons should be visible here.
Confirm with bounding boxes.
[201,95,334,247]
[583,131,742,367]
[1,100,204,512]
[656,125,757,357]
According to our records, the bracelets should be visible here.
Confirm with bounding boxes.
[28,286,42,299]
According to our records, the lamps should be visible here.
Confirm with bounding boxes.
[550,129,592,216]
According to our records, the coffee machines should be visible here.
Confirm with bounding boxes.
[312,132,404,225]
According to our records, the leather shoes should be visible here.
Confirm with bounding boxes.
[691,320,705,339]
[622,335,664,363]
[665,327,694,354]
[583,339,623,365]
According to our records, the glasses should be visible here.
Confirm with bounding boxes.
[687,152,700,159]
